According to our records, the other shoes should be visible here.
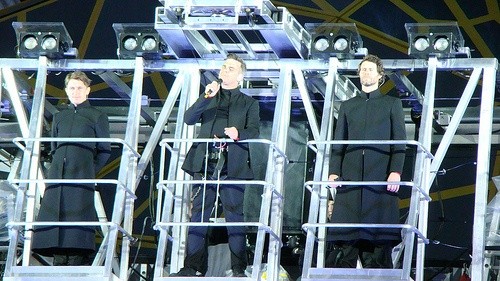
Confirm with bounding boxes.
[233,266,247,276]
[168,266,203,277]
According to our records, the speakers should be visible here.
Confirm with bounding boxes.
[241,119,317,233]
[94,122,174,261]
[396,146,497,265]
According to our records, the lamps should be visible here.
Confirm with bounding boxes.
[11,21,73,58]
[404,22,471,60]
[112,23,167,60]
[306,22,367,59]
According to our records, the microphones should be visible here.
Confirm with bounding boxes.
[204,79,223,98]
[325,177,343,189]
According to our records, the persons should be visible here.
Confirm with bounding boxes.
[327,54,406,269]
[31,72,111,266]
[169,53,261,277]
